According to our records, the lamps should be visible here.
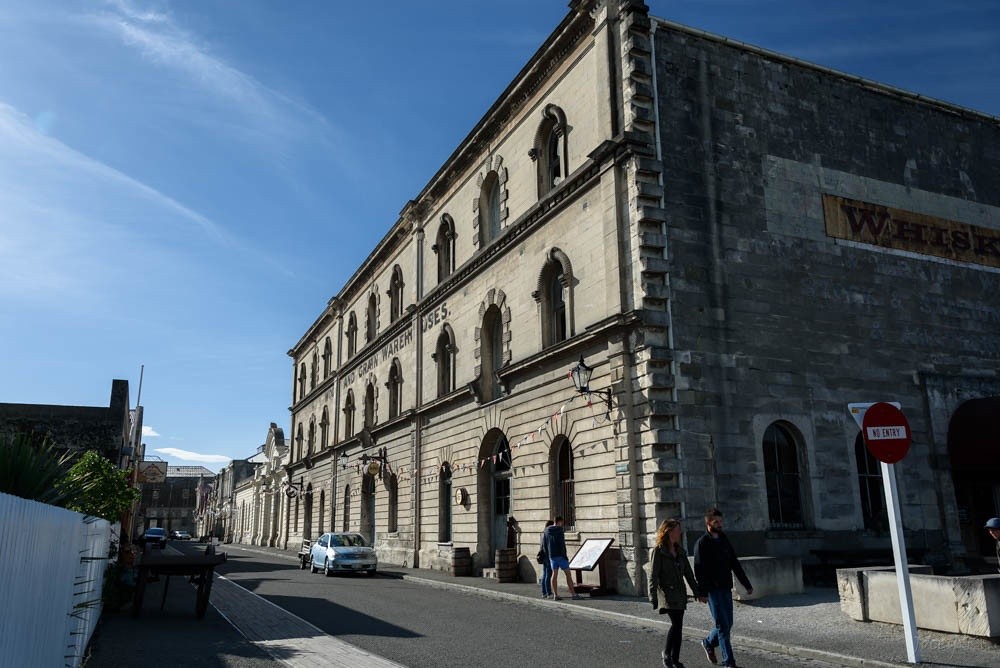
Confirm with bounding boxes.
[339,450,360,473]
[261,482,267,492]
[571,352,612,409]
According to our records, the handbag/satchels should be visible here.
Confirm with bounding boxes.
[537,550,546,564]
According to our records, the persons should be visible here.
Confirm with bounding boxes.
[647,517,701,668]
[544,516,586,601]
[539,520,560,600]
[694,508,755,668]
[985,518,1000,574]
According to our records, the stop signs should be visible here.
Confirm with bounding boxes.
[861,401,912,464]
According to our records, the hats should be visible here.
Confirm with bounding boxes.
[984,518,1000,528]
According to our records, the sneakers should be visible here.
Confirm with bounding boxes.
[672,661,686,668]
[572,594,584,600]
[662,648,672,668]
[542,592,547,598]
[701,640,717,664]
[724,663,740,668]
[554,596,559,600]
[547,593,554,599]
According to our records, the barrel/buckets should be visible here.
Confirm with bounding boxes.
[453,547,469,577]
[495,548,517,584]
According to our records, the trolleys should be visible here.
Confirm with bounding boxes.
[131,537,227,619]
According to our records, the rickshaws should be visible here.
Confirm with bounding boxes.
[297,539,317,569]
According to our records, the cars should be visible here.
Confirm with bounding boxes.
[309,532,378,577]
[173,530,192,541]
[142,528,167,549]
